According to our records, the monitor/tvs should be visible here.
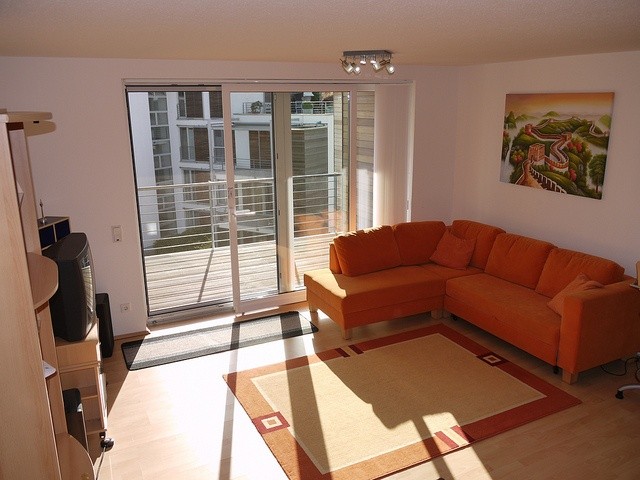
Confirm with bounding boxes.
[42,233,97,342]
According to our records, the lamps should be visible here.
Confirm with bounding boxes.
[340,50,396,76]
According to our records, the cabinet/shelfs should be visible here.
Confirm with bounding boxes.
[55,318,107,435]
[120,311,318,370]
[38,216,70,254]
[1,110,96,480]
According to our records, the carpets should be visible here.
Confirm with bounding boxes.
[222,322,583,480]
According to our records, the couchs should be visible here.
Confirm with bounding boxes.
[304,219,640,385]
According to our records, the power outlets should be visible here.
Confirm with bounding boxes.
[120,303,131,313]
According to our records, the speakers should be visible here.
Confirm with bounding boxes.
[95,293,114,358]
[62,388,89,452]
